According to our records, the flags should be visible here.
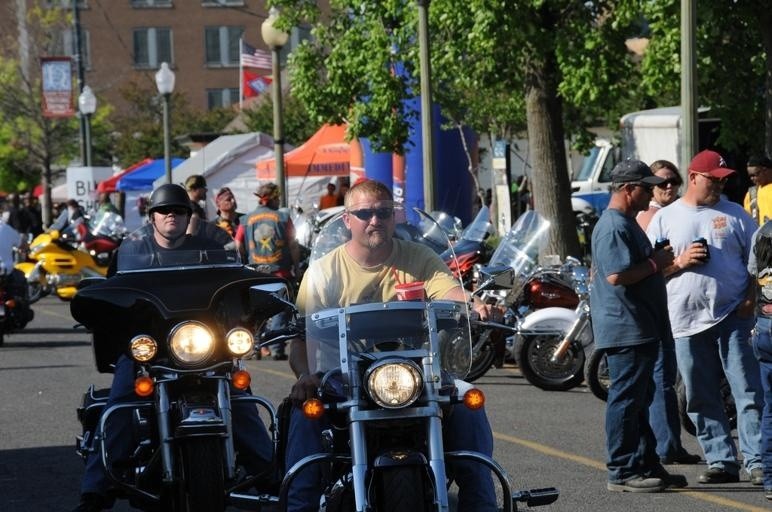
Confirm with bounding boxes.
[240,40,273,70]
[242,70,272,96]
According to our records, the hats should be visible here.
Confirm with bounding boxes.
[611,157,665,187]
[688,149,735,180]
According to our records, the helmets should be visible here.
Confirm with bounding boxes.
[147,183,194,213]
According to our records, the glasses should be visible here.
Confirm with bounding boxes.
[655,177,683,190]
[152,206,188,216]
[748,169,762,178]
[345,207,397,222]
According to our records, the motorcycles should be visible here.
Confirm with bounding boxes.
[435,209,589,384]
[72,215,290,511]
[0,219,36,346]
[256,202,559,512]
[15,207,123,301]
[516,260,589,393]
[440,204,495,287]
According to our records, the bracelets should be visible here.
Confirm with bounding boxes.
[647,256,658,273]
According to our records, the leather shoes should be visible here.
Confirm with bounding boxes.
[746,468,765,484]
[765,488,772,500]
[605,475,666,493]
[696,468,741,484]
[661,451,702,466]
[647,468,688,489]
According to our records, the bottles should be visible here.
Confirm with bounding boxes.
[693,237,711,262]
[656,238,670,250]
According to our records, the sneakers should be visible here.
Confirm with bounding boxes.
[242,352,262,361]
[271,351,289,361]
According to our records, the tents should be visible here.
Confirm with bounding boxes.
[152,132,296,225]
[50,184,69,203]
[117,159,184,194]
[255,120,350,207]
[97,158,150,194]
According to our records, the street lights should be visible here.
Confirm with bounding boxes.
[153,60,176,182]
[77,85,98,167]
[259,6,296,207]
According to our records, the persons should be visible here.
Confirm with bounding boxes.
[748,221,771,498]
[95,193,120,216]
[185,174,209,238]
[645,149,759,487]
[319,182,338,211]
[742,152,772,229]
[337,183,350,206]
[590,159,688,493]
[512,175,532,221]
[0,220,30,282]
[283,180,498,512]
[636,160,683,231]
[233,183,302,362]
[1,190,41,262]
[78,185,279,512]
[68,199,86,225]
[208,187,246,240]
